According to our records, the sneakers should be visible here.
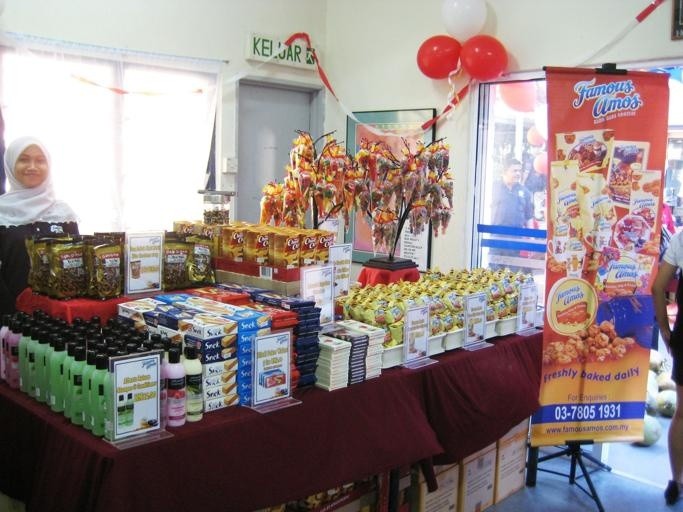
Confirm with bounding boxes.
[664,481,683,506]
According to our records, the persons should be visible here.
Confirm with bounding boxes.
[490,141,547,312]
[651,226,683,506]
[0,135,81,315]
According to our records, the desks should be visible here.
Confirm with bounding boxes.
[1,262,543,512]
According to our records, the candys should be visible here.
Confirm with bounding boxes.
[262,135,454,245]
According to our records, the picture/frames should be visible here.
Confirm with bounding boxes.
[342,107,436,274]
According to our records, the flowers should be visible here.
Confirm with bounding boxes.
[258,128,452,262]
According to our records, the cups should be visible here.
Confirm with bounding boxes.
[548,228,609,281]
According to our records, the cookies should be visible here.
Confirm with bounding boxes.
[220,323,238,405]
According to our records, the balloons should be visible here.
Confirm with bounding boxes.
[442,0,488,44]
[459,34,506,82]
[497,81,538,114]
[526,126,544,148]
[415,34,462,81]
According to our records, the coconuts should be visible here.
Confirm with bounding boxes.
[637,349,677,446]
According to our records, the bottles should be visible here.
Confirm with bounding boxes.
[1,308,204,444]
